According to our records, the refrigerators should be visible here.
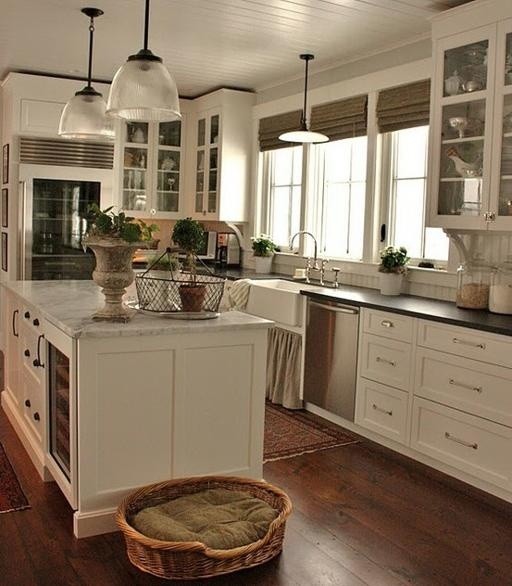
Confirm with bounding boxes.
[13,164,114,281]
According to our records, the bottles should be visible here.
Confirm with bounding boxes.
[488,255,512,314]
[455,251,489,309]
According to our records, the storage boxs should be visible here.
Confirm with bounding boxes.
[2,279,277,539]
[302,287,512,507]
[421,2,512,232]
[1,71,122,281]
[117,88,255,222]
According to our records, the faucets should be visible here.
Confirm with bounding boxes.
[288,231,340,287]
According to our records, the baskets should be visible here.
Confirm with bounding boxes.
[135,247,225,314]
[112,472,292,579]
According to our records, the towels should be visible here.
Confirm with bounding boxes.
[222,275,254,314]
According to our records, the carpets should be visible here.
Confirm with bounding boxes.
[263,396,363,466]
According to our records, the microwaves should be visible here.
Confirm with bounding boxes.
[172,231,217,260]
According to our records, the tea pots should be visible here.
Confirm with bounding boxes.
[462,80,483,92]
[444,70,463,96]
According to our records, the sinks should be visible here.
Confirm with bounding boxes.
[250,278,315,293]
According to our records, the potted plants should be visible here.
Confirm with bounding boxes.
[145,251,180,312]
[83,203,162,319]
[377,245,410,294]
[252,234,276,272]
[171,217,209,311]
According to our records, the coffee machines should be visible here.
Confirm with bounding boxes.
[216,232,241,267]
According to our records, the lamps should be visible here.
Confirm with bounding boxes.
[57,8,118,142]
[105,0,182,124]
[275,52,330,145]
